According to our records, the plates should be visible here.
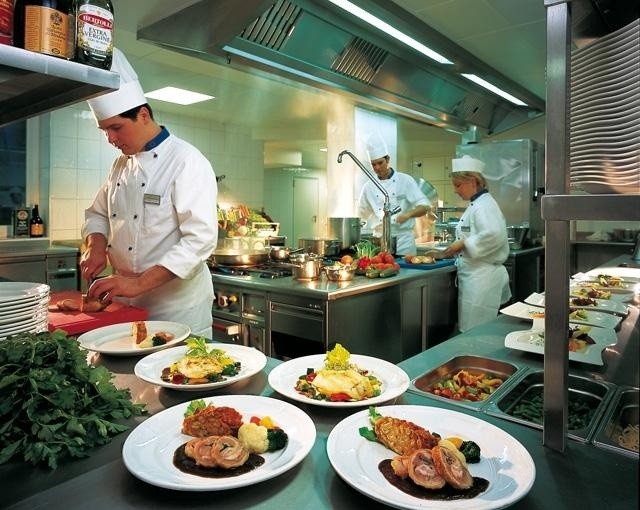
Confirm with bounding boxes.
[566,16,639,195]
[498,272,639,366]
[0,282,51,343]
[327,405,537,509]
[78,320,192,357]
[267,353,411,407]
[123,395,317,492]
[133,343,267,389]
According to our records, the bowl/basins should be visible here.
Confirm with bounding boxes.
[324,265,357,281]
[507,225,530,251]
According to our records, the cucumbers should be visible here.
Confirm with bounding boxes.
[366,268,398,277]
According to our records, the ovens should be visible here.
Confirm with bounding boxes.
[210,316,272,345]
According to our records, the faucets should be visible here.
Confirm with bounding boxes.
[338,150,402,251]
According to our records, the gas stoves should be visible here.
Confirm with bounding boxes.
[206,264,293,325]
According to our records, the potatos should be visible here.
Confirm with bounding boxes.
[342,256,353,265]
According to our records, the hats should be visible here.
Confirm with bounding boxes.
[84,47,148,120]
[451,158,484,173]
[365,130,388,160]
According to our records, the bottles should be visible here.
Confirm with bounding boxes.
[28,204,44,238]
[0,1,115,71]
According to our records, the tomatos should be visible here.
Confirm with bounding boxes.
[358,252,400,271]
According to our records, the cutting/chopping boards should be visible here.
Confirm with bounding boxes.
[394,257,456,270]
[47,289,149,337]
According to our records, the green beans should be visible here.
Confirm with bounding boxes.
[512,392,593,429]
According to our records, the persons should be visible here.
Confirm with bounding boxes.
[352,137,432,257]
[441,155,515,338]
[78,47,219,341]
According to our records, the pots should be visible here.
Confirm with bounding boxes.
[212,250,268,265]
[327,217,368,253]
[296,237,342,257]
[278,261,318,281]
[270,246,305,261]
[613,228,638,242]
